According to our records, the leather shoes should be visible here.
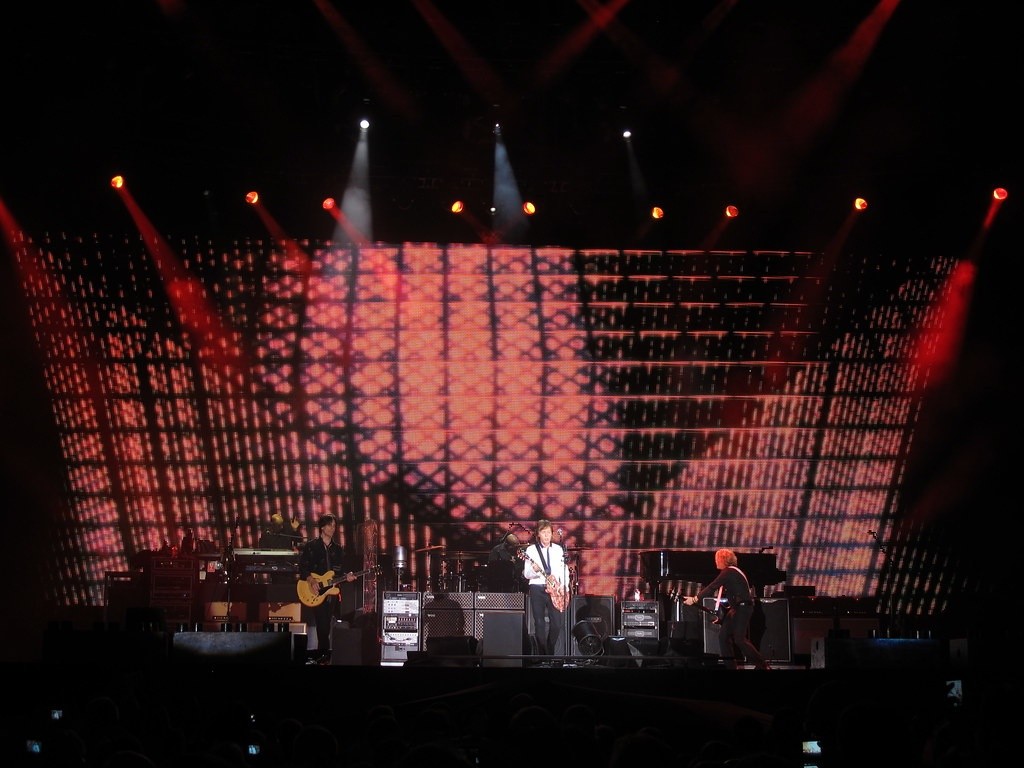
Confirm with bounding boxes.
[754,661,768,671]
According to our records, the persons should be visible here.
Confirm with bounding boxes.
[300,514,356,650]
[523,520,564,656]
[683,549,767,669]
[489,533,520,593]
[0,664,1024,767]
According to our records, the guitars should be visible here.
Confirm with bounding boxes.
[297,568,372,606]
[671,588,727,628]
[517,549,571,610]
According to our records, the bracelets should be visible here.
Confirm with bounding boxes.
[693,597,696,603]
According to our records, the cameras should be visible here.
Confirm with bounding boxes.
[945,680,962,707]
[802,741,821,753]
[804,764,818,768]
[249,744,261,755]
[26,740,42,753]
[50,710,63,720]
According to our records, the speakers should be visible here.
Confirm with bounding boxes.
[703,598,938,670]
[406,592,661,667]
[174,631,292,666]
[330,626,364,666]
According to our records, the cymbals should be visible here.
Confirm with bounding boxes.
[412,546,447,552]
[444,551,477,560]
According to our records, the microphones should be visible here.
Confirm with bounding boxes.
[557,529,562,536]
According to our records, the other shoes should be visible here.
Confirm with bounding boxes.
[539,657,553,667]
[317,648,333,664]
